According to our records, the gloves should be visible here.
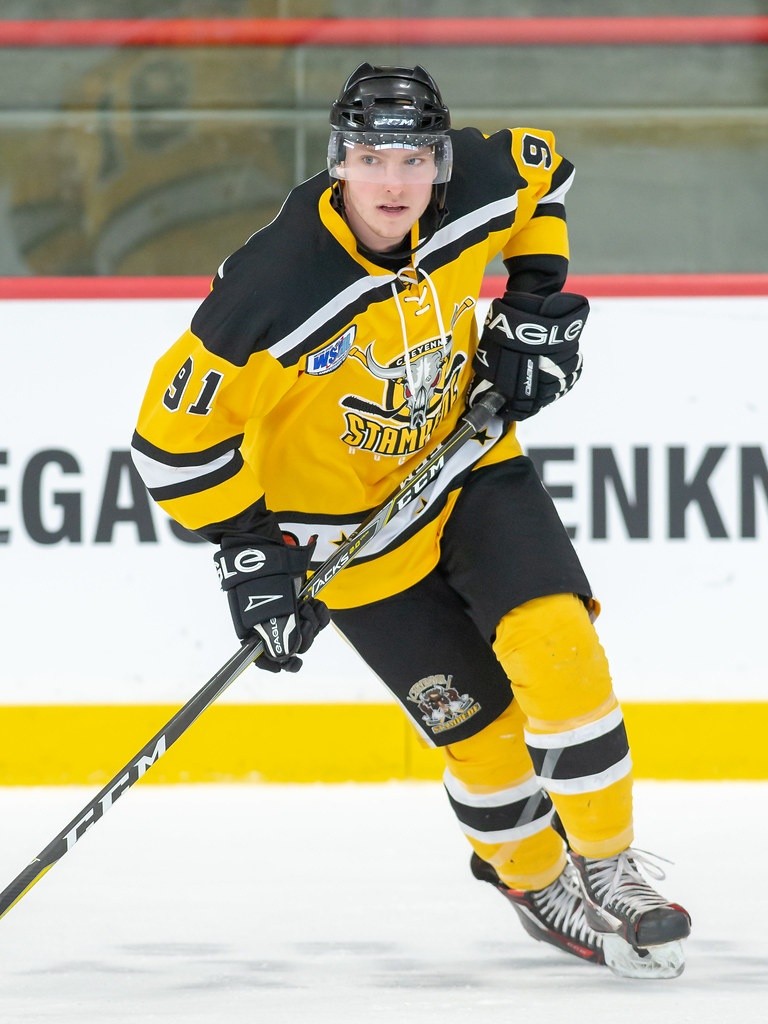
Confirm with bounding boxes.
[465,291,590,421]
[213,532,330,673]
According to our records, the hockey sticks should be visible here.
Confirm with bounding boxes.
[1,387,508,913]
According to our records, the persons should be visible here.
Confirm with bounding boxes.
[128,62,696,984]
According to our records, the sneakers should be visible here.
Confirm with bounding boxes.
[471,810,692,979]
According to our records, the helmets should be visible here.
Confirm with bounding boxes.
[327,62,453,185]
